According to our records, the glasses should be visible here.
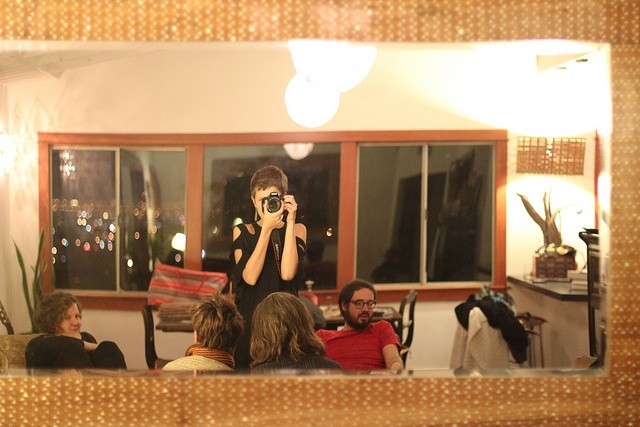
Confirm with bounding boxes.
[348,298,377,310]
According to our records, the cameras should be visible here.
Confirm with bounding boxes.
[260,192,288,215]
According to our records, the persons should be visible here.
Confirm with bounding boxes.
[312,280,411,376]
[159,294,243,375]
[26,292,128,374]
[250,289,347,376]
[226,167,309,373]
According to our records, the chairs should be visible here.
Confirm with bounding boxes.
[399,289,417,368]
[141,306,176,368]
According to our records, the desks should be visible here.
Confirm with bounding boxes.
[156,307,401,329]
